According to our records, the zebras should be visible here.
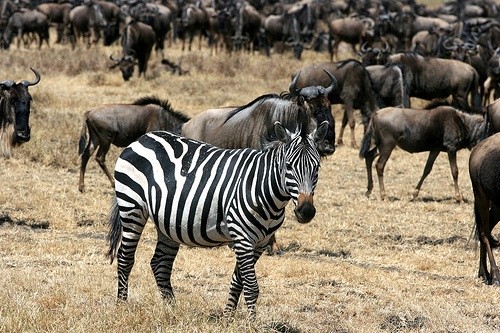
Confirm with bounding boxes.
[106,121,330,321]
